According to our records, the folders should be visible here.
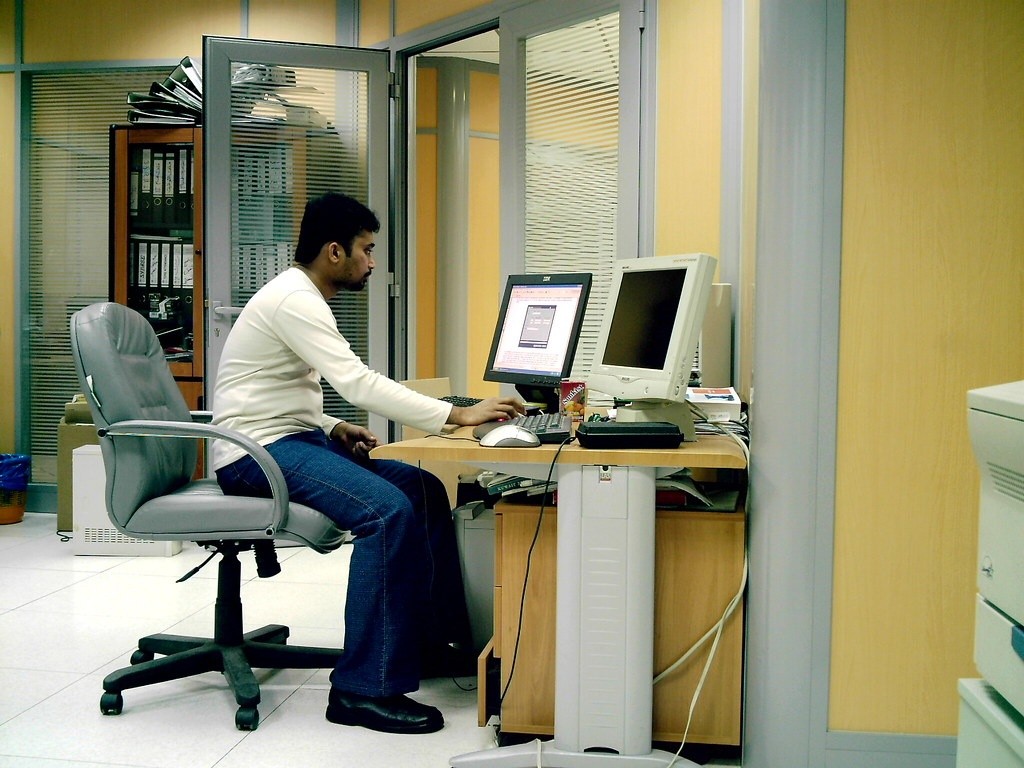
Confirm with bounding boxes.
[128,144,294,324]
[126,55,327,125]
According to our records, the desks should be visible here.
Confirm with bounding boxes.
[368,406,747,768]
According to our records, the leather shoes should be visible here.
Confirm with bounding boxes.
[326,684,444,734]
[418,645,478,680]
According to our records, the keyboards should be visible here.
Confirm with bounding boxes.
[437,395,485,408]
[473,410,571,445]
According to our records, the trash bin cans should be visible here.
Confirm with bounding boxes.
[0,453,32,525]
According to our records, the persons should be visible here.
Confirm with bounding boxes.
[211,194,527,734]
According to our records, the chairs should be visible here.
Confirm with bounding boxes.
[69,302,352,732]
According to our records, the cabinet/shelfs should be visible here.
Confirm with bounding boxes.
[109,123,312,483]
[478,502,746,759]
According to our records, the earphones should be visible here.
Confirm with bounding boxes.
[333,249,341,258]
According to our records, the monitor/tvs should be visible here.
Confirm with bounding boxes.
[585,253,718,442]
[481,272,593,415]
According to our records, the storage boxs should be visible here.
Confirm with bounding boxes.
[58,416,99,534]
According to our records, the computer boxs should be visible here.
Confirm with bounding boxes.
[72,443,182,558]
[454,502,495,651]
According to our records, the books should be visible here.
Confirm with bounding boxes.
[487,467,714,507]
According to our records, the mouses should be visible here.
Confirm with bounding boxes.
[479,424,542,448]
